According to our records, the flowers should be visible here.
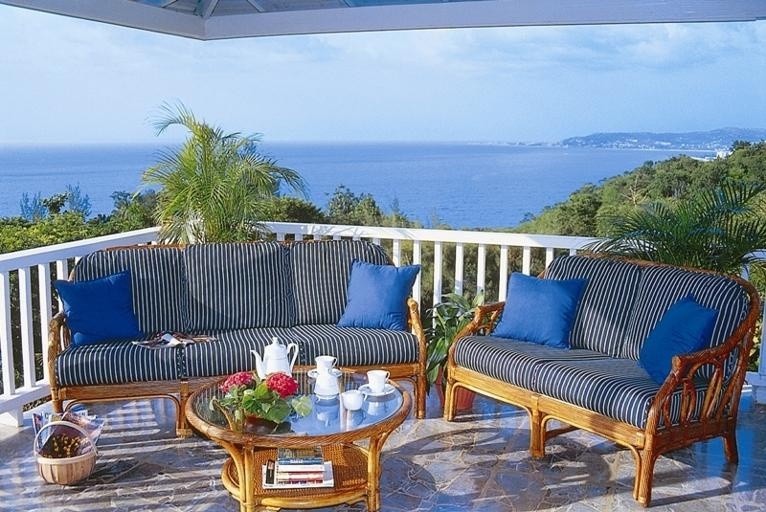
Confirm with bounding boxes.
[209,372,300,425]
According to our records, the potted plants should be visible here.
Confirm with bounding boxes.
[424,289,495,411]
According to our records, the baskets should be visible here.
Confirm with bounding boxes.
[33,418,97,485]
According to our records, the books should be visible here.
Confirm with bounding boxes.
[276,446,326,472]
[274,465,324,484]
[260,457,335,490]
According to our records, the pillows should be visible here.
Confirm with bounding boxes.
[489,256,750,386]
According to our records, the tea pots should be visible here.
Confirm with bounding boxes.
[248,337,298,380]
[314,368,339,402]
[341,389,369,411]
[312,400,340,427]
[342,410,366,428]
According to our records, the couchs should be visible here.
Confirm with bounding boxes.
[444,255,760,508]
[49,240,426,438]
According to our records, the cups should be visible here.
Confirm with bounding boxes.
[367,401,388,416]
[367,369,391,392]
[315,355,337,375]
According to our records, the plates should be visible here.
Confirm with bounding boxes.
[307,368,343,379]
[359,383,395,397]
